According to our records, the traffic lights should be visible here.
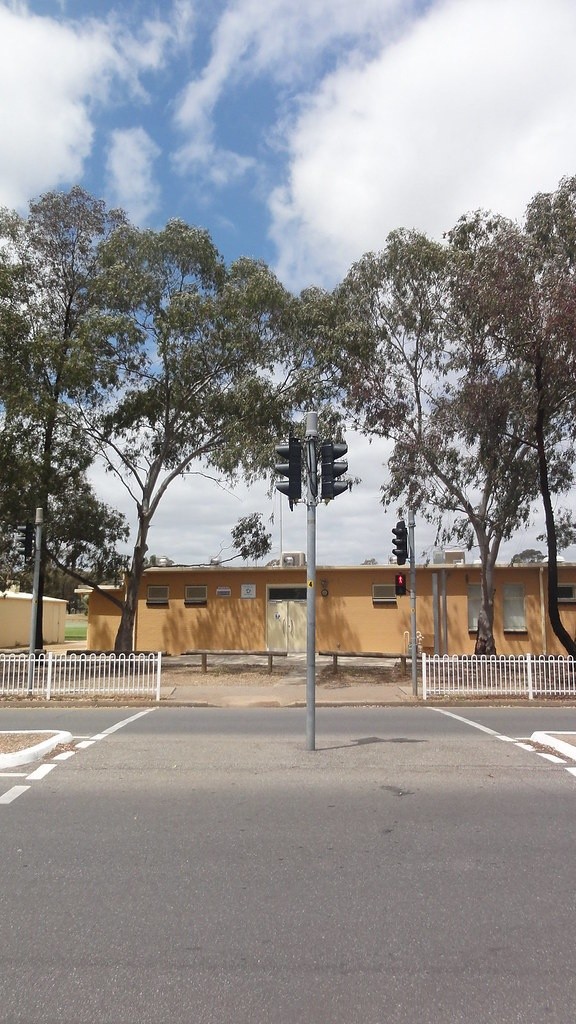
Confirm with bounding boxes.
[321,442,348,500]
[395,574,406,595]
[392,521,407,565]
[16,521,32,562]
[274,432,301,511]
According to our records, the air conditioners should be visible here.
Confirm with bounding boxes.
[281,551,305,567]
[445,551,465,565]
